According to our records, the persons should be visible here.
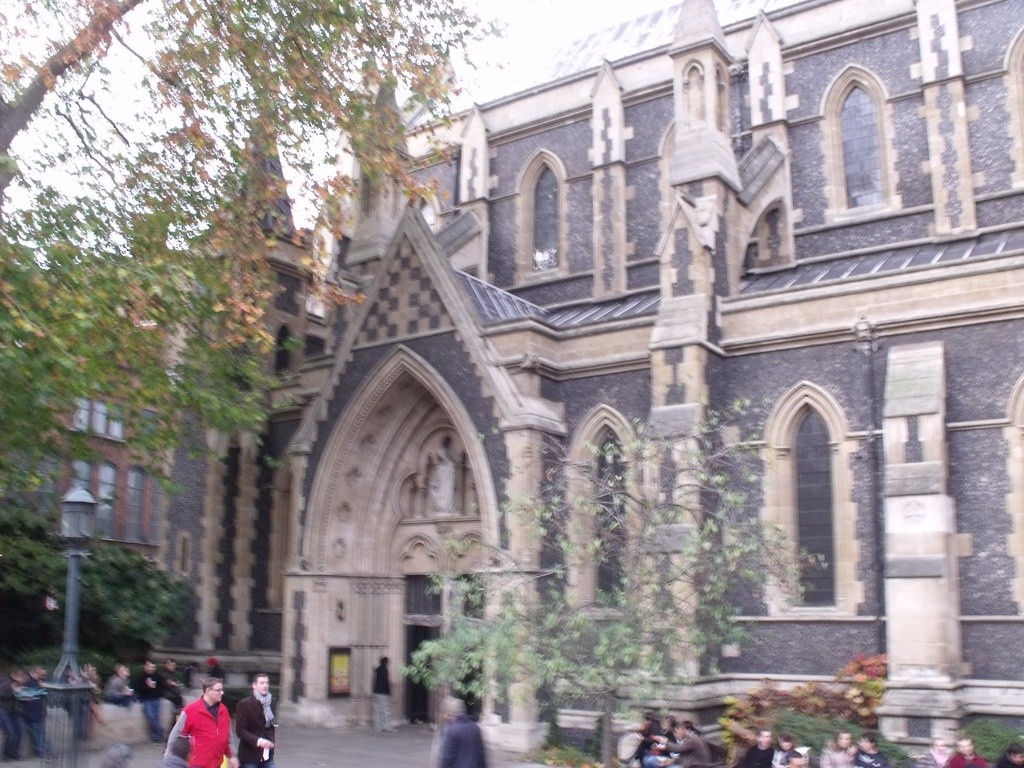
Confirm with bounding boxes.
[997,743,1024,768]
[617,711,709,768]
[80,663,107,727]
[105,665,134,709]
[428,448,460,517]
[235,674,276,768]
[372,657,398,732]
[164,678,239,768]
[202,657,226,693]
[433,696,485,768]
[916,736,987,768]
[134,658,183,743]
[732,729,802,768]
[0,666,59,761]
[819,729,889,768]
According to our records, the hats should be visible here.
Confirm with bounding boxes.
[440,695,466,717]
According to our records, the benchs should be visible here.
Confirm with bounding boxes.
[0,695,199,758]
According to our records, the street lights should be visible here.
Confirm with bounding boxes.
[51,483,99,683]
